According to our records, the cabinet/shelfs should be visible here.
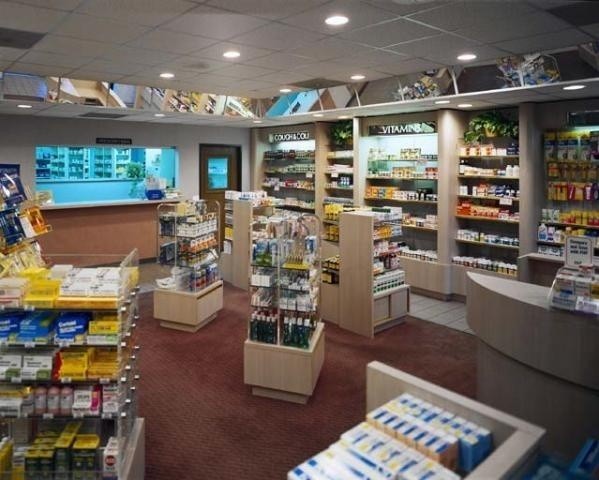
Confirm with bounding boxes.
[321,206,409,339]
[285,359,547,479]
[218,194,272,292]
[262,152,598,303]
[242,214,325,406]
[151,199,223,334]
[1,247,147,480]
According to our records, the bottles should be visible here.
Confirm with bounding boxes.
[250,306,318,349]
[373,268,405,296]
[192,269,207,291]
[322,254,339,284]
